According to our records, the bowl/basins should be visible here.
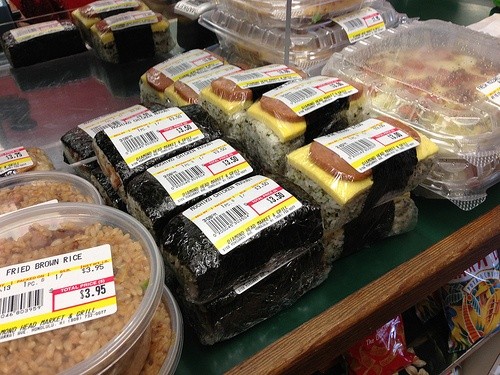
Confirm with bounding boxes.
[138,283,185,375]
[0,201,166,374]
[0,170,105,205]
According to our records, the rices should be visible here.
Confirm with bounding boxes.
[0,174,173,375]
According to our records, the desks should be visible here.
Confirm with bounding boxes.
[0,13,500,375]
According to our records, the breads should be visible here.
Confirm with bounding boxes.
[0,0,500,342]
